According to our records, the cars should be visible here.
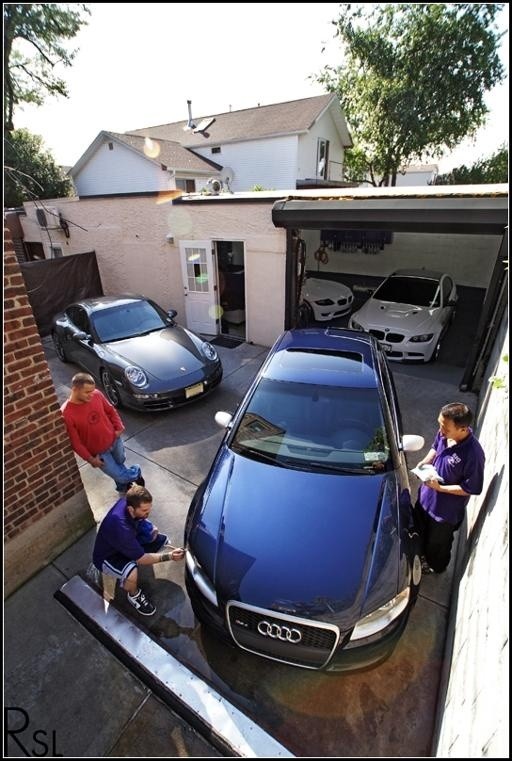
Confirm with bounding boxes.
[184,266,460,676]
[51,294,223,413]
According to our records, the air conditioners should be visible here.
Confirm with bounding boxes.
[33,206,63,231]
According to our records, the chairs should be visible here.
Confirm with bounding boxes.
[333,396,371,432]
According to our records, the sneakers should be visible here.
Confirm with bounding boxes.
[421,563,447,574]
[136,476,145,486]
[127,587,157,616]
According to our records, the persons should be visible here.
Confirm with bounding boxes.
[61,372,145,494]
[93,485,186,616]
[412,401,486,573]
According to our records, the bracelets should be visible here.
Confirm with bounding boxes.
[160,552,169,562]
